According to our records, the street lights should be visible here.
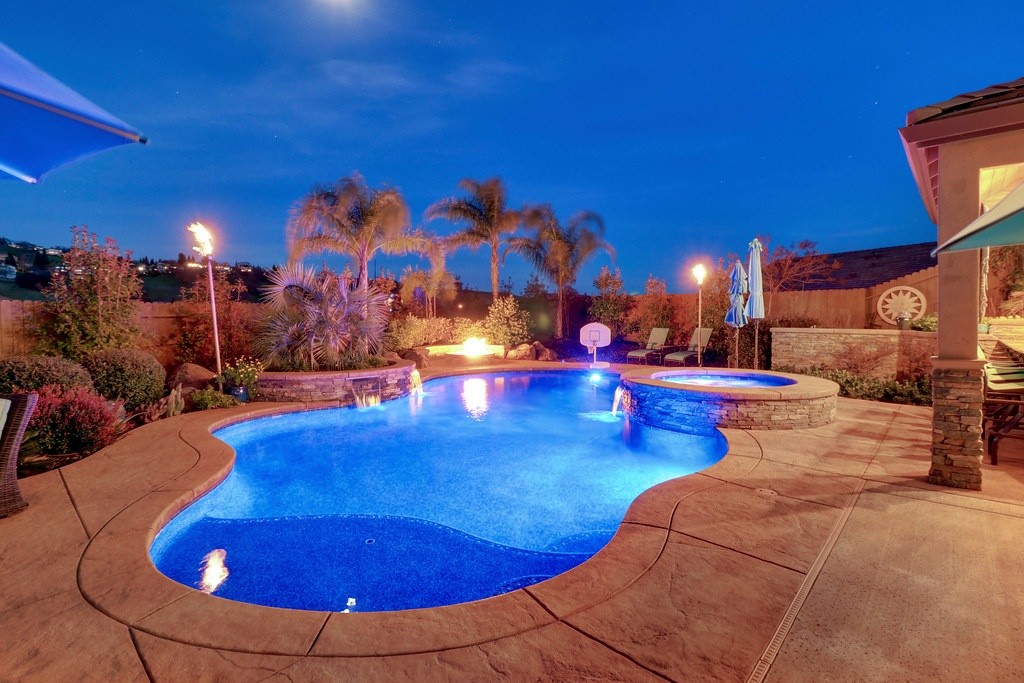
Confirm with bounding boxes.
[187,223,224,391]
[693,265,707,365]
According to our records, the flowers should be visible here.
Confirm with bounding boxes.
[896,310,913,322]
[213,355,265,387]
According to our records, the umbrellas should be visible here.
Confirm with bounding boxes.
[724,260,748,368]
[743,238,765,370]
[928,179,1024,257]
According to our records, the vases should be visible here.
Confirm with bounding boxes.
[896,318,912,330]
[227,387,249,403]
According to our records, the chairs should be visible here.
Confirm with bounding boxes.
[664,328,714,367]
[977,339,1024,465]
[0,393,39,518]
[626,327,670,365]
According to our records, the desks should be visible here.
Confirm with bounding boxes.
[652,345,687,365]
[990,325,1024,354]
